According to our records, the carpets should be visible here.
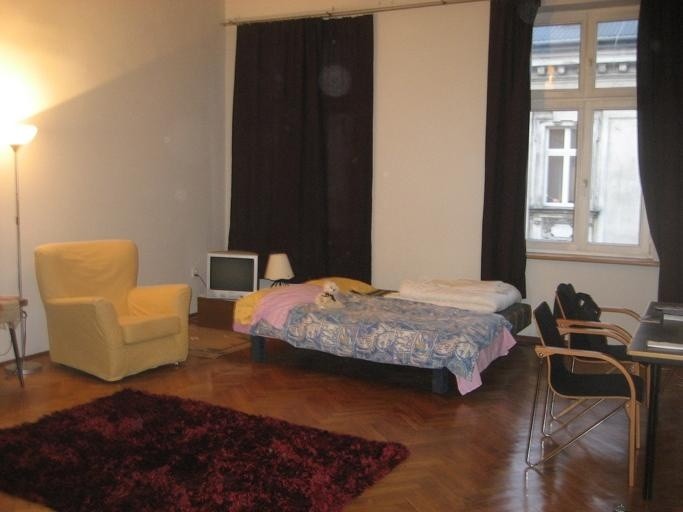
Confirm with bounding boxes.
[2,385,413,512]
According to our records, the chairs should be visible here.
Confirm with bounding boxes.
[33,239,193,383]
[524,301,646,489]
[550,282,643,421]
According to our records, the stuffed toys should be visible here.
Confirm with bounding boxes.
[315,281,340,308]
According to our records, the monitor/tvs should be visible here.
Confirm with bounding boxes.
[206,251,259,300]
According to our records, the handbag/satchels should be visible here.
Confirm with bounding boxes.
[570,293,608,352]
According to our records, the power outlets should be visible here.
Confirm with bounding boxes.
[193,269,200,277]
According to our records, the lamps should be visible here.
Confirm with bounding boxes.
[262,252,295,286]
[0,121,44,374]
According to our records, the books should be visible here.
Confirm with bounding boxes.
[664,308,683,321]
[647,330,683,350]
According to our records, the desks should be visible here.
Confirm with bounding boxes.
[624,298,683,511]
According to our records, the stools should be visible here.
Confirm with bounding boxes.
[0,296,27,386]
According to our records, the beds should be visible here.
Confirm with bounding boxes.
[232,273,534,395]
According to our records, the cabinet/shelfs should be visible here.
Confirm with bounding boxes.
[196,296,234,332]
